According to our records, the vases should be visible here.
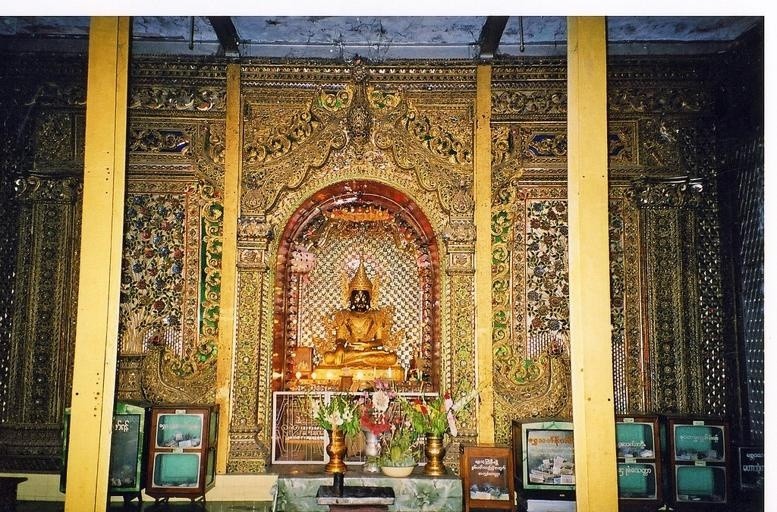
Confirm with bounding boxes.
[360,430,382,472]
[424,433,446,477]
[324,429,348,474]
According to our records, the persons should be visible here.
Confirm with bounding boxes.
[321,262,397,368]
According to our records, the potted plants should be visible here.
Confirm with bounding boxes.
[362,428,422,477]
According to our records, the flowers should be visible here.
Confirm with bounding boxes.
[308,376,484,436]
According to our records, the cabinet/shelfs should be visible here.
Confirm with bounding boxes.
[460,416,576,512]
[615,412,734,512]
[145,405,220,511]
